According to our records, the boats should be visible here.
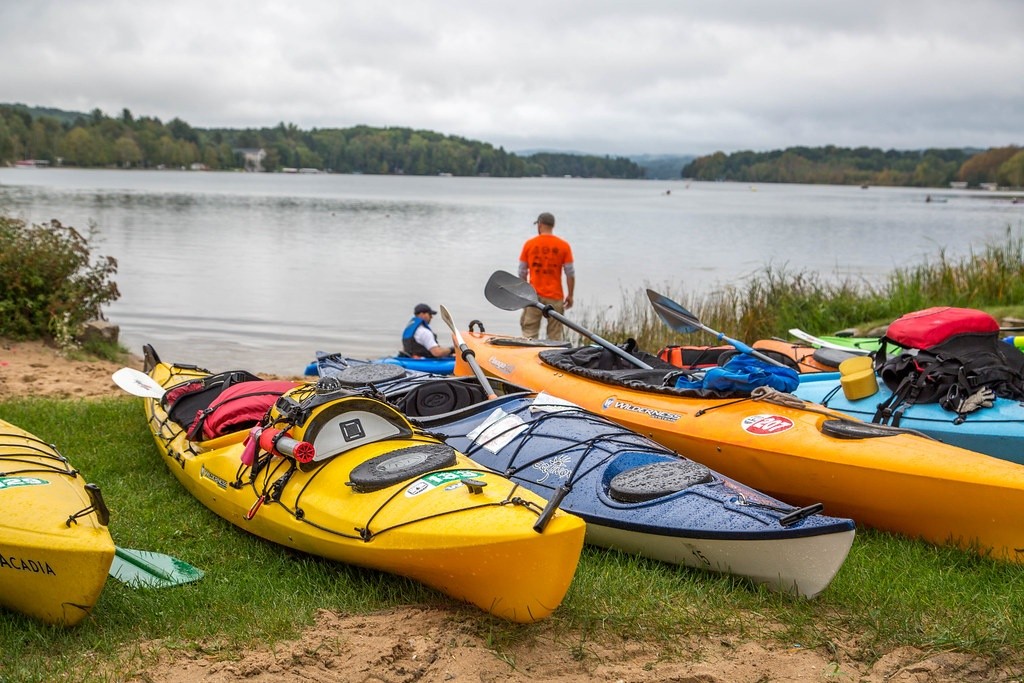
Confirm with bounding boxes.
[306,353,456,375]
[0,419,116,626]
[143,343,587,623]
[304,351,857,600]
[453,331,1022,566]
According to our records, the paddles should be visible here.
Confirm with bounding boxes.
[112,544,206,592]
[483,269,654,369]
[110,367,166,399]
[787,327,871,354]
[644,288,800,375]
[438,304,499,400]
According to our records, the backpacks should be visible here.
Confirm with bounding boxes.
[872,335,1024,427]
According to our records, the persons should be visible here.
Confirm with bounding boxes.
[518,213,575,342]
[402,304,455,360]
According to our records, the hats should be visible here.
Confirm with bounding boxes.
[534,213,554,225]
[415,304,437,314]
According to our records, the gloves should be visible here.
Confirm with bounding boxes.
[947,384,997,414]
[751,386,806,409]
